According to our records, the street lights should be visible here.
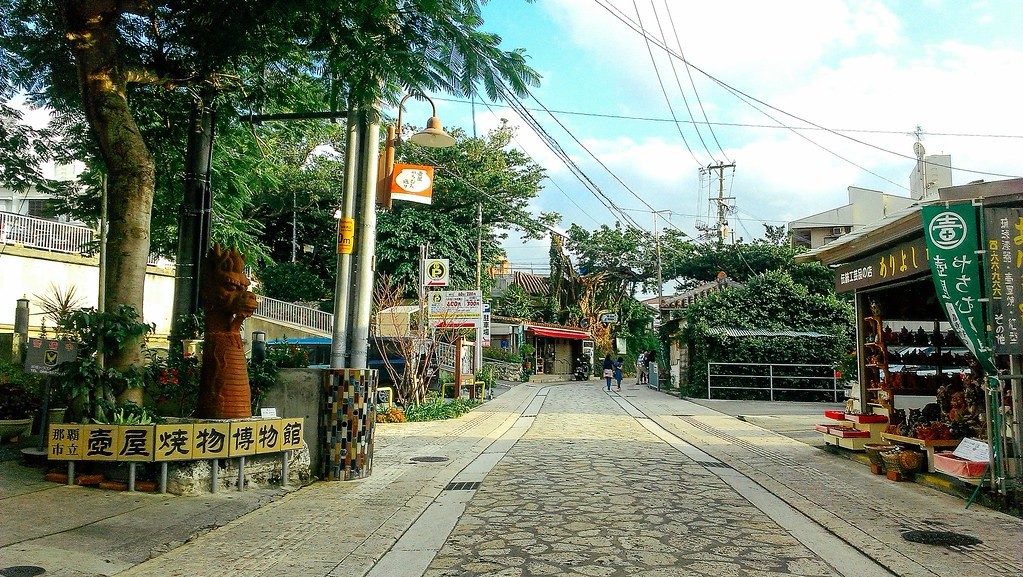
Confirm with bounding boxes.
[349,91,456,369]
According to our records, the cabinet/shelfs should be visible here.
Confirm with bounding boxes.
[863,340,995,398]
[812,402,891,449]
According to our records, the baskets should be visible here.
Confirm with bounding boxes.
[879,451,916,474]
[863,443,905,466]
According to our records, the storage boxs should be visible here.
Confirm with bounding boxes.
[934,452,990,479]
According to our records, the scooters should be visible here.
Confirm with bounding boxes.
[568,358,590,383]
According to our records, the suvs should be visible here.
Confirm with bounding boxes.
[367,358,452,404]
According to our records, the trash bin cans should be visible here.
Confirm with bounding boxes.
[843,383,853,402]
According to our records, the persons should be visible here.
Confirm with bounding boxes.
[636,349,656,385]
[603,354,624,392]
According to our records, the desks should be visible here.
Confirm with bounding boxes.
[878,431,965,472]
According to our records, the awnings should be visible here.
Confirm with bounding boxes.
[528,326,586,339]
